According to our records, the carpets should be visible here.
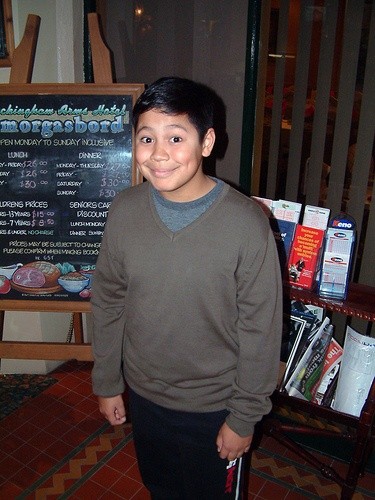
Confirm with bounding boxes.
[0,374,58,421]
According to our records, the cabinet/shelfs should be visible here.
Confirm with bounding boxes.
[253,281,375,500]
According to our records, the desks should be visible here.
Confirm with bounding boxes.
[0,298,96,362]
